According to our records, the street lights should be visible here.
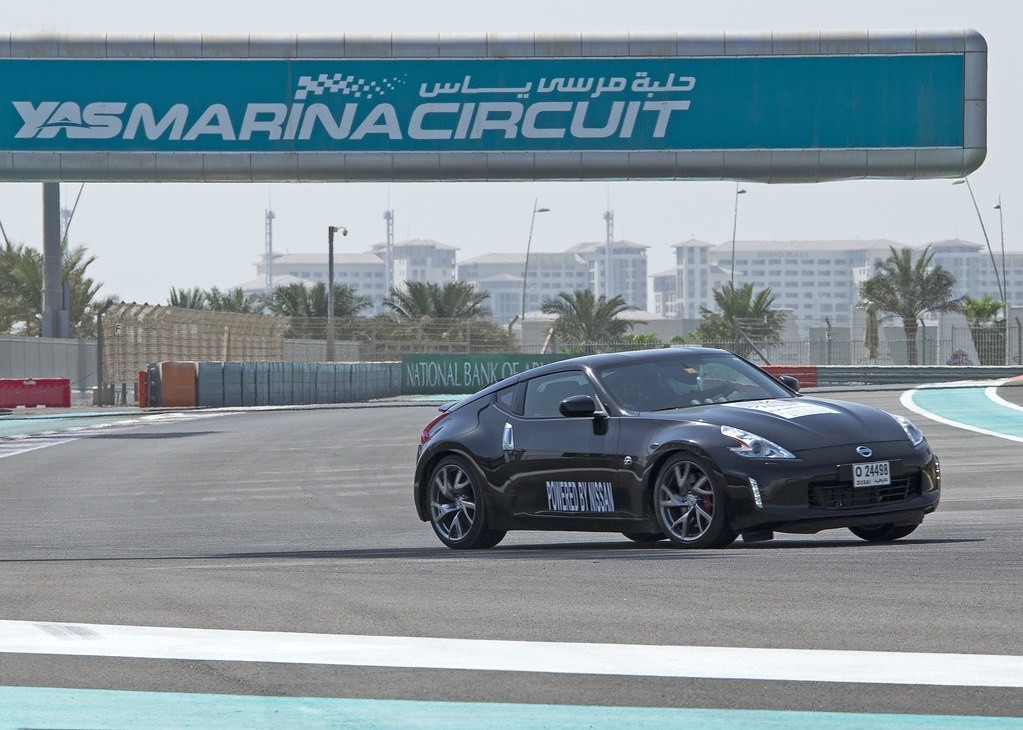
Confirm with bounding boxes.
[952,176,1005,315]
[730,184,748,289]
[521,196,551,320]
[992,194,1007,307]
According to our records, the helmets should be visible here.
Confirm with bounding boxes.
[669,360,703,396]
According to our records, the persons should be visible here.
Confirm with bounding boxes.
[650,365,744,410]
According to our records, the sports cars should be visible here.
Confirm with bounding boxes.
[410,348,943,551]
